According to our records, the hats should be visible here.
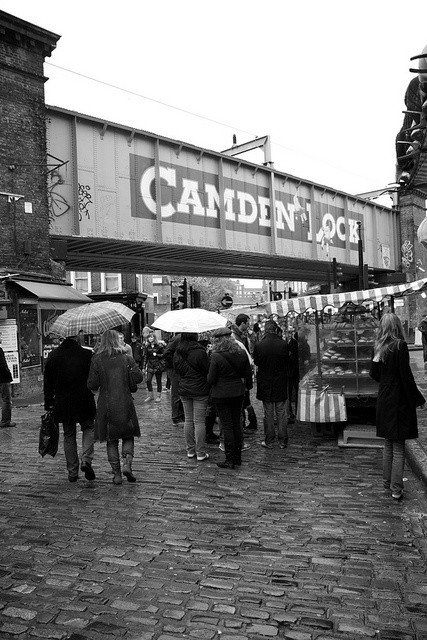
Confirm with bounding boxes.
[214,327,232,337]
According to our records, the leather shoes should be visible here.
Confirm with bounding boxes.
[80,461,95,480]
[217,460,234,469]
[68,475,78,483]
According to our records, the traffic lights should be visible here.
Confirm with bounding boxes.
[274,292,281,300]
[288,287,297,299]
[172,298,179,310]
[363,263,377,290]
[178,282,186,309]
[334,266,343,289]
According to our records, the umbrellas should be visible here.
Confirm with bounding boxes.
[48,300,137,340]
[151,308,228,334]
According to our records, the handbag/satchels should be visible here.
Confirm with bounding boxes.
[38,411,58,457]
[157,360,165,372]
[128,370,137,392]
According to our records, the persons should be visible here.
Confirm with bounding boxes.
[172,332,210,461]
[43,335,96,483]
[253,320,260,333]
[229,324,256,366]
[252,320,290,449]
[235,312,251,356]
[206,326,253,470]
[0,332,16,428]
[142,332,166,404]
[286,326,310,419]
[417,314,427,366]
[129,332,144,372]
[118,332,133,359]
[368,313,425,501]
[205,331,223,444]
[87,329,144,486]
[198,331,212,347]
[231,336,257,430]
[262,326,296,426]
[167,334,186,427]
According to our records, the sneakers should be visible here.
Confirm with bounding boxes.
[278,442,285,449]
[218,442,226,452]
[5,422,16,427]
[261,441,274,448]
[241,444,251,451]
[188,451,196,458]
[197,453,209,461]
[392,489,403,498]
[207,439,220,444]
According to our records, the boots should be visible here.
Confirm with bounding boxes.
[144,391,154,402]
[245,415,257,429]
[122,453,136,482]
[155,392,161,402]
[110,462,123,484]
